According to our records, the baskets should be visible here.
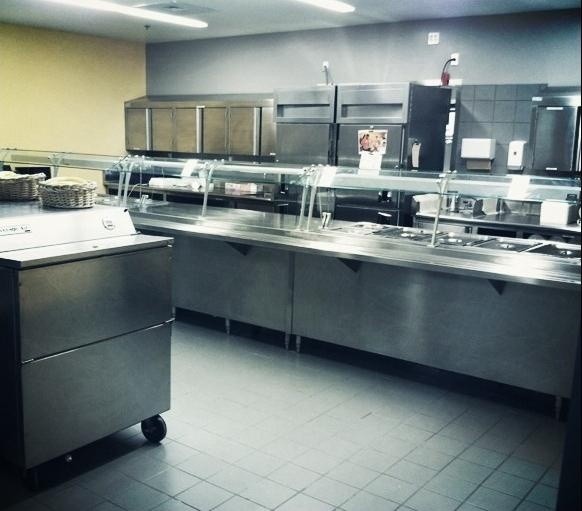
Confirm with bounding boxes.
[38,177,98,209]
[0,173,44,201]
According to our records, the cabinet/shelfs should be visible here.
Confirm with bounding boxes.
[533,106,581,174]
[202,106,260,156]
[154,105,200,153]
[126,107,150,152]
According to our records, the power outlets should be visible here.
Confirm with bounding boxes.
[450,53,460,67]
[321,61,329,73]
